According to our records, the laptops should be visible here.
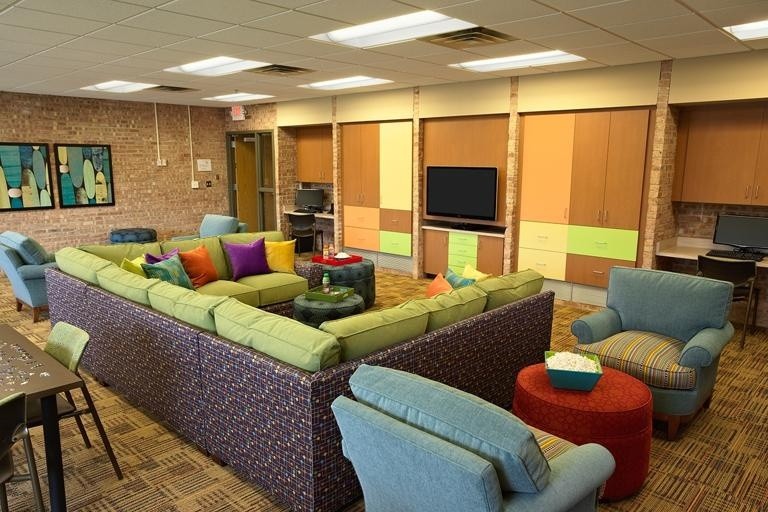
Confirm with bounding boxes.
[293,189,325,214]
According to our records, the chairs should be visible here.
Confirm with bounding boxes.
[695,253,761,348]
[0,392,45,512]
[16,321,124,480]
[283,212,324,258]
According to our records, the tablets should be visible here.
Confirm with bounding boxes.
[712,212,768,251]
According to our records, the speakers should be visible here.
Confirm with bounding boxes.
[331,203,334,215]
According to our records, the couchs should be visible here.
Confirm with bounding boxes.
[171,214,247,241]
[0,230,58,323]
[331,363,616,512]
[45,231,555,512]
[570,265,735,441]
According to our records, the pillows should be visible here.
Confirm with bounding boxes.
[264,240,297,274]
[445,269,474,290]
[120,254,147,278]
[177,244,220,289]
[145,248,179,264]
[141,254,196,292]
[224,237,271,281]
[424,273,454,299]
[461,264,491,283]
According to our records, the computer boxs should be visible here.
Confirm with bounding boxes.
[288,234,316,254]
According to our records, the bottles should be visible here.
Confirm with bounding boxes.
[329,244,334,259]
[323,244,329,260]
[322,270,330,294]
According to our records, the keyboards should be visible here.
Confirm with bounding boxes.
[705,249,767,262]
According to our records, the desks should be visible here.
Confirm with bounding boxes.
[283,209,334,219]
[656,236,768,278]
[0,322,84,512]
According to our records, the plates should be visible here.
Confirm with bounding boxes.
[333,251,351,259]
[312,254,362,268]
[305,281,355,303]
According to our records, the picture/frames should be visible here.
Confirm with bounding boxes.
[53,143,115,209]
[0,142,55,211]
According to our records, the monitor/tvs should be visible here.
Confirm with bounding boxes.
[422,163,500,230]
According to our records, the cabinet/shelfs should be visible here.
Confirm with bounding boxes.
[421,229,504,279]
[519,113,575,224]
[517,221,638,288]
[569,110,652,231]
[671,104,768,206]
[342,205,413,257]
[341,121,413,211]
[296,126,333,184]
